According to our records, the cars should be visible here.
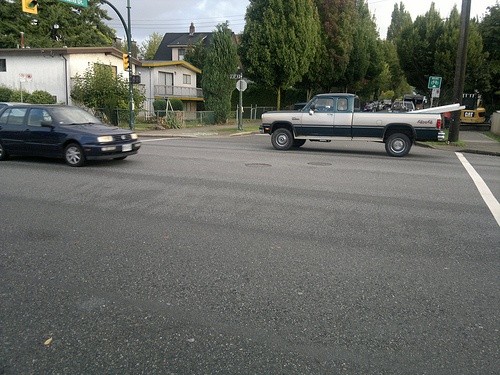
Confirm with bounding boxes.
[0,102,142,166]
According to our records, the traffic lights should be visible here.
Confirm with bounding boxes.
[22,0,38,14]
[123,53,130,71]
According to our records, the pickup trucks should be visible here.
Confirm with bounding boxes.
[260,93,443,156]
